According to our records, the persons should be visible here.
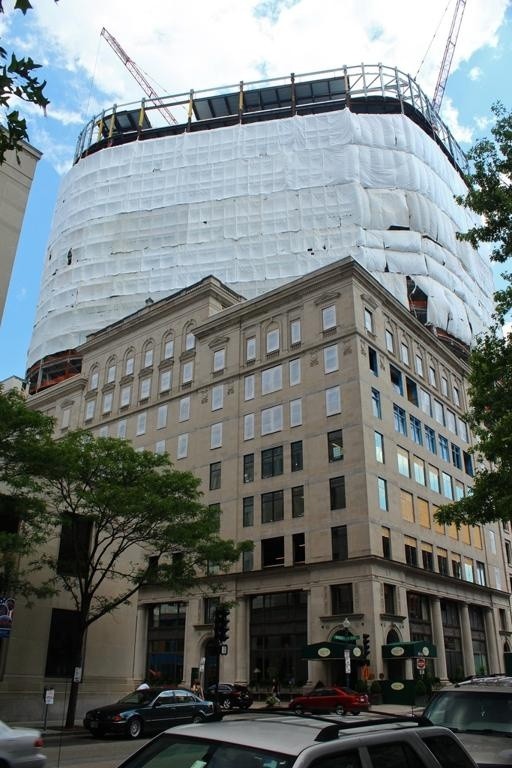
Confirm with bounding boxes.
[193,680,206,701]
[147,664,162,685]
[272,675,278,698]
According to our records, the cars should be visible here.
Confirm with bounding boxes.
[288,686,370,717]
[0,720,49,767]
[83,687,216,739]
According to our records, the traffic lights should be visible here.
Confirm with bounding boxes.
[364,634,370,657]
[219,605,231,642]
[220,644,228,655]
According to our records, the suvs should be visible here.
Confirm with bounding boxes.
[203,683,255,710]
[116,707,480,767]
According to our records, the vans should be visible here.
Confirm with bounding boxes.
[421,672,512,768]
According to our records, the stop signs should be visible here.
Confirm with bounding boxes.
[417,659,425,669]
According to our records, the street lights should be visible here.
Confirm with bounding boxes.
[341,616,351,687]
